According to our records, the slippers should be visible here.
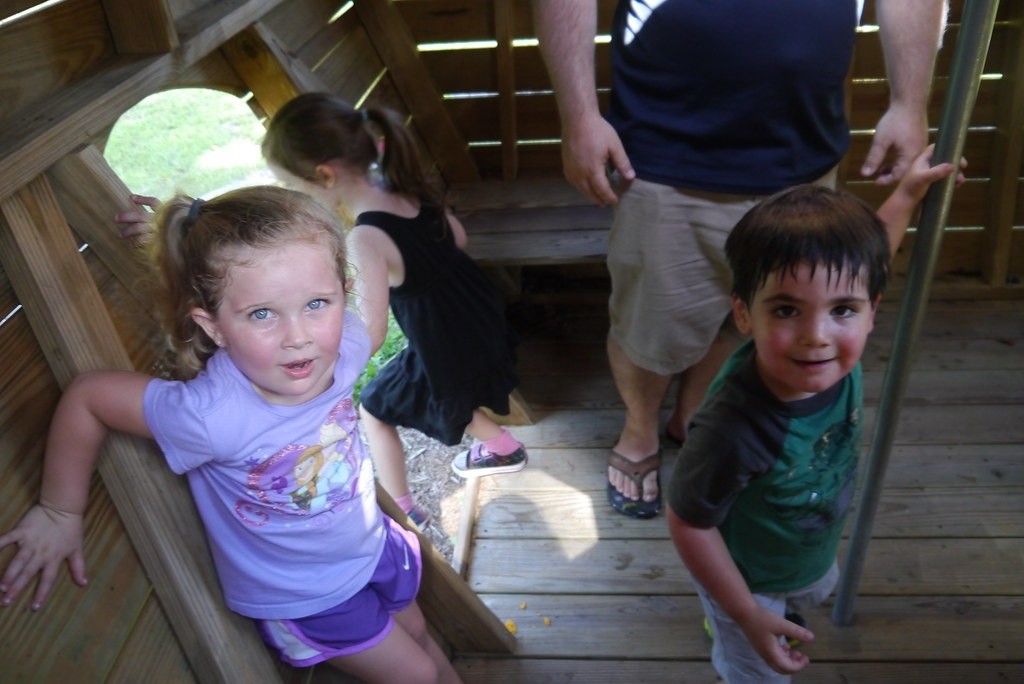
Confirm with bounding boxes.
[606,443,663,520]
[661,429,685,448]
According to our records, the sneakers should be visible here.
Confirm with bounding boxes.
[408,505,432,531]
[450,443,525,477]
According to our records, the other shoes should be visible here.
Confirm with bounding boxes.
[702,610,806,648]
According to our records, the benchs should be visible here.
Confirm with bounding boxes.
[444,177,632,269]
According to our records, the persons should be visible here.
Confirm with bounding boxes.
[2,184,465,684]
[533,1,950,518]
[665,143,968,684]
[114,93,528,479]
